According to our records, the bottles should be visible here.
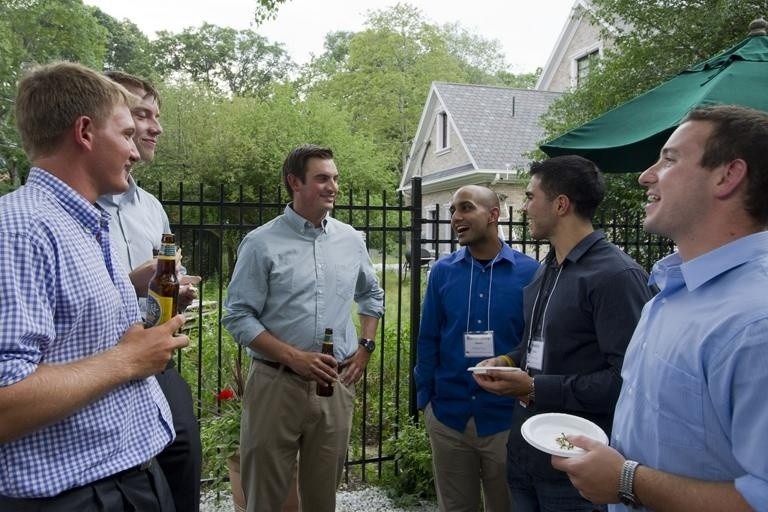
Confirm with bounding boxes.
[144,234,179,328]
[316,328,334,397]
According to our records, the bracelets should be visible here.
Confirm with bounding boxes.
[529,376,536,402]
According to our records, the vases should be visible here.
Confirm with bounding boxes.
[226,449,302,512]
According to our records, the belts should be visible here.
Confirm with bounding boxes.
[252,356,346,375]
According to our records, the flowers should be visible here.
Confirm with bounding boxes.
[186,352,242,495]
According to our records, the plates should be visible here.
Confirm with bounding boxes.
[467,366,521,375]
[520,413,609,458]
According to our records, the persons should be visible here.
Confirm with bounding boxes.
[412,183,542,512]
[93,70,201,511]
[551,102,768,512]
[472,154,659,510]
[0,61,190,511]
[222,144,384,512]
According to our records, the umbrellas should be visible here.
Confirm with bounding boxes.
[537,19,768,176]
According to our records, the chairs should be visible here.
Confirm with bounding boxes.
[400,248,432,281]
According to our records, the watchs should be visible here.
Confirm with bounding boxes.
[358,338,375,353]
[617,460,641,509]
